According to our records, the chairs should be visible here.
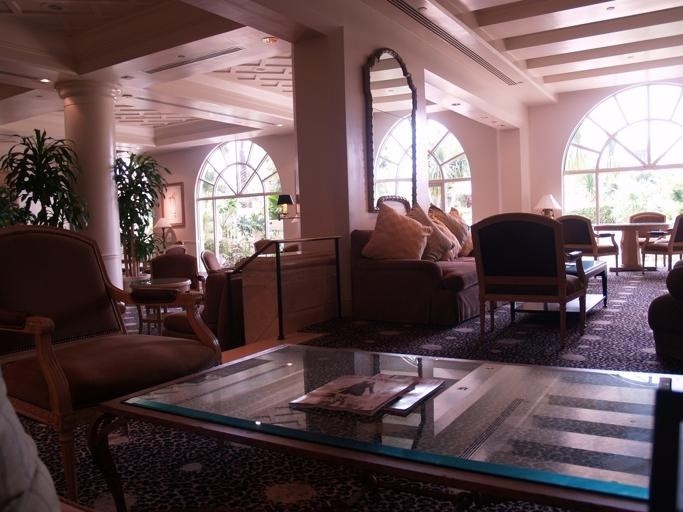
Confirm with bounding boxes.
[130,246,223,337]
[0,226,221,500]
[470,211,683,348]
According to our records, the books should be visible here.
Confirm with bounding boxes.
[381,378,447,416]
[287,374,415,418]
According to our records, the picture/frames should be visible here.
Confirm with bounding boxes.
[160,182,186,226]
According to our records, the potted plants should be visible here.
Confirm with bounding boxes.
[114,152,173,293]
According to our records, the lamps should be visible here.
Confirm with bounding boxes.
[534,194,562,220]
[277,194,293,215]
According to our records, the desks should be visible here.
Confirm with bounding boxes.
[86,342,683,511]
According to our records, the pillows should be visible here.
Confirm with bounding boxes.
[362,202,473,261]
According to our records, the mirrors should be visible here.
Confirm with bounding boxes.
[363,47,416,213]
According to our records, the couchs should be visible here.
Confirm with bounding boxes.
[351,228,509,322]
